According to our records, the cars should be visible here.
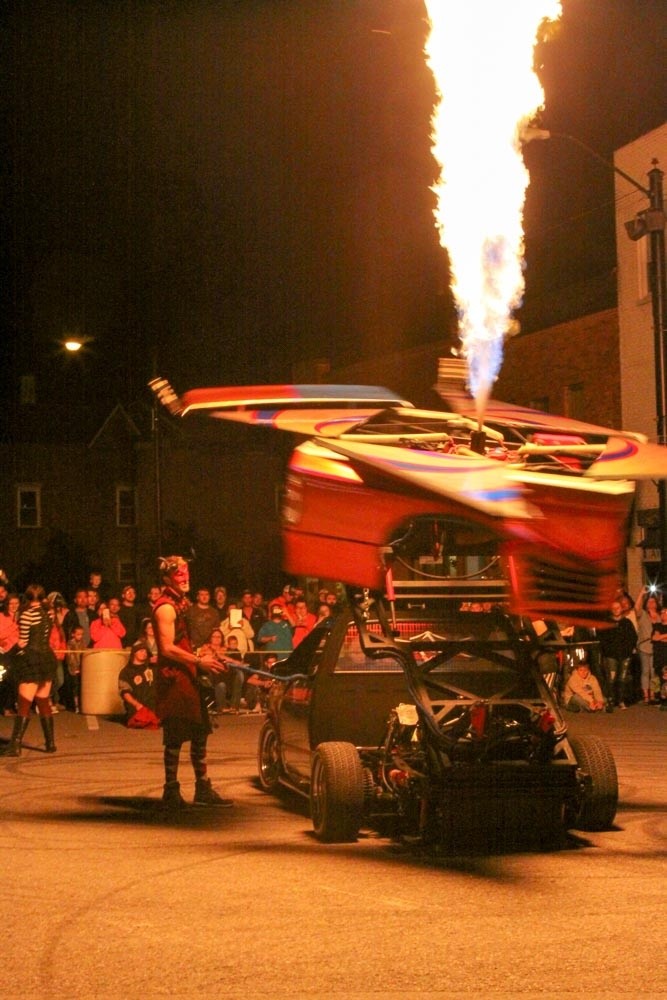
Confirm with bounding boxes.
[256,597,620,846]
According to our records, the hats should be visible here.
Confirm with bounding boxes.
[131,641,146,654]
[215,587,226,595]
[242,589,252,596]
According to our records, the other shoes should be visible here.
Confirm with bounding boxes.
[50,705,59,714]
[75,705,80,714]
[606,698,667,713]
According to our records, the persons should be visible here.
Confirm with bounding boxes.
[151,555,234,811]
[0,585,58,756]
[0,567,369,728]
[541,581,667,712]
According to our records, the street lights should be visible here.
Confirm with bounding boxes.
[517,124,667,610]
[66,334,164,556]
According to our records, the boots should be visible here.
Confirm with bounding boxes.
[193,777,234,807]
[40,716,57,753]
[0,715,29,756]
[160,782,193,812]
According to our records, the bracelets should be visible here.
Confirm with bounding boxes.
[195,656,201,669]
